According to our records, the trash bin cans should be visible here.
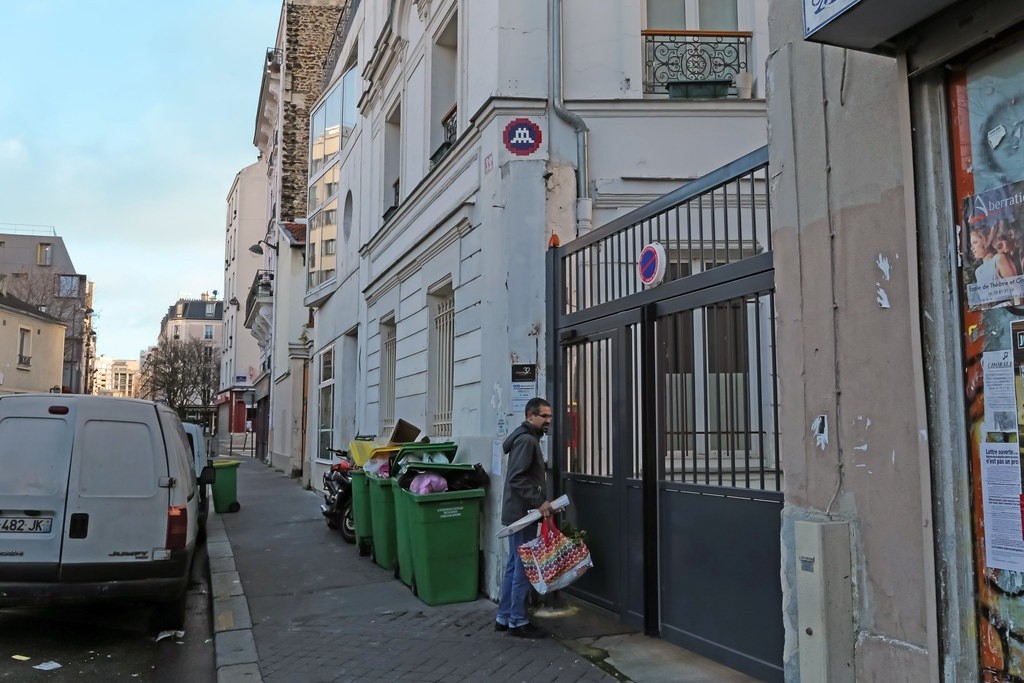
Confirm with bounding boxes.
[390,441,458,587]
[349,441,387,557]
[365,445,403,570]
[211,460,240,513]
[402,462,486,607]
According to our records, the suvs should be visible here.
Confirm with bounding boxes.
[0,392,216,630]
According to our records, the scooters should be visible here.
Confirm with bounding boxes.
[320,448,356,545]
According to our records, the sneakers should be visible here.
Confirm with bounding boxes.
[495,621,510,632]
[509,623,547,638]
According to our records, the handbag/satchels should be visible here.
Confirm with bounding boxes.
[517,514,593,594]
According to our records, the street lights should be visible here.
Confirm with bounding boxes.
[69,303,95,392]
[83,327,96,395]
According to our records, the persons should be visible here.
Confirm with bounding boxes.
[497,397,558,639]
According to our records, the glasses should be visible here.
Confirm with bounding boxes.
[533,413,553,421]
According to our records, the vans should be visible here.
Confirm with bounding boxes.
[181,422,214,547]
